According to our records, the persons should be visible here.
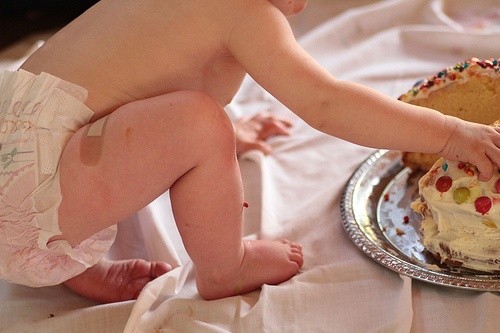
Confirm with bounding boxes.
[0,0,500,306]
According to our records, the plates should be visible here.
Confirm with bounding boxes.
[340,148,500,293]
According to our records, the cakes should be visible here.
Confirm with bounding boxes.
[397,56,500,170]
[410,120,500,274]
